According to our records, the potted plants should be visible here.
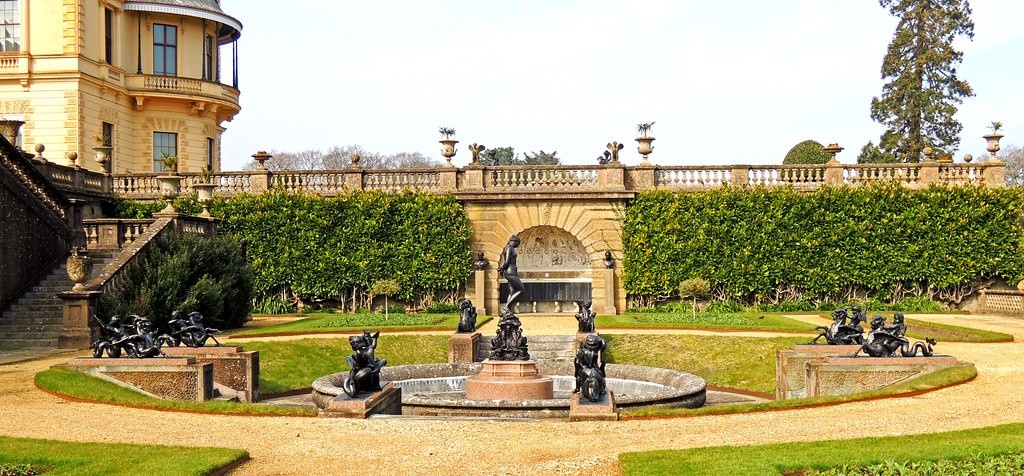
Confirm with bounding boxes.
[155,153,183,212]
[91,134,113,173]
[439,127,459,167]
[983,122,1004,162]
[192,167,219,217]
[635,121,655,166]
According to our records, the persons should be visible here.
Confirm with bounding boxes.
[94,314,145,358]
[168,311,197,348]
[473,252,489,269]
[602,252,615,268]
[498,236,524,316]
[123,167,135,193]
[469,142,486,162]
[882,313,907,357]
[846,305,862,345]
[607,140,624,160]
